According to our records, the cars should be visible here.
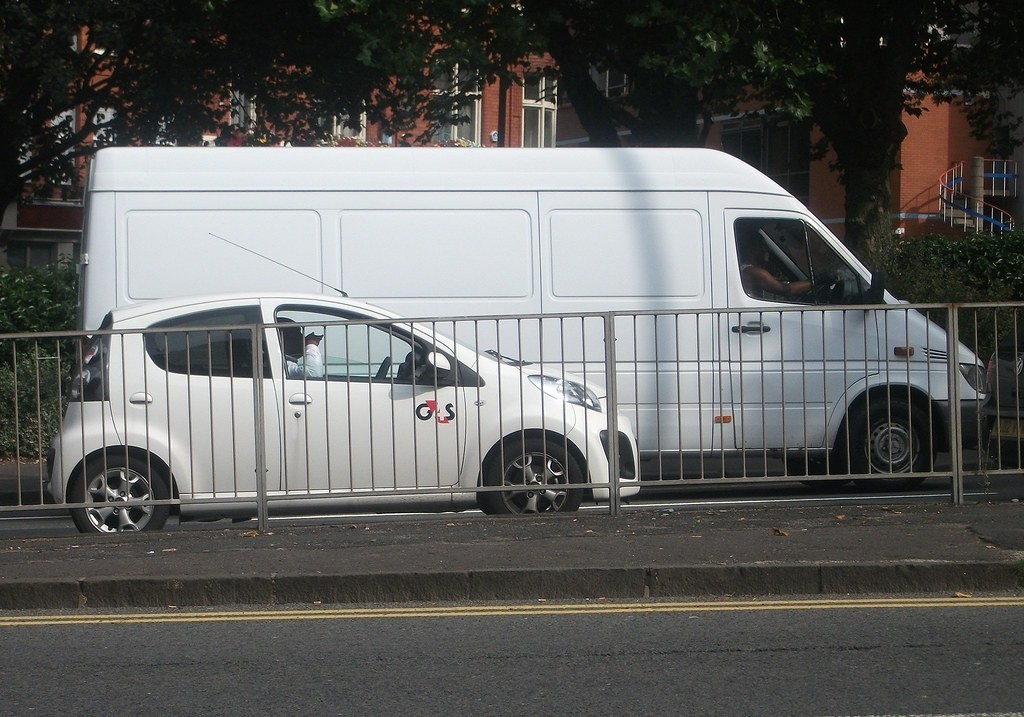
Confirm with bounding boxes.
[41,291,643,534]
[984,331,1024,469]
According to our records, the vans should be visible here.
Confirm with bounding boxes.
[74,146,991,490]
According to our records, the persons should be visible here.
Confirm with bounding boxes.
[275,317,324,378]
[739,232,839,301]
